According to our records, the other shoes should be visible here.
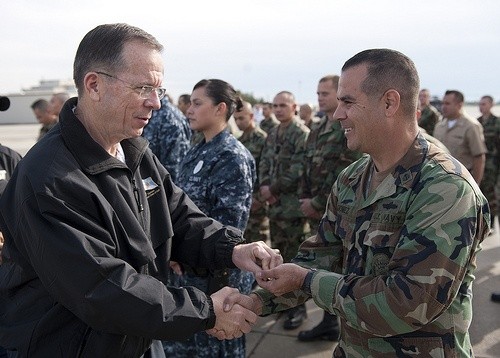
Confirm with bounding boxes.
[283,302,307,329]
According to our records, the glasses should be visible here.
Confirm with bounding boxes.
[96,72,166,100]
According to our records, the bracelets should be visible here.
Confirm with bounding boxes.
[302,269,318,297]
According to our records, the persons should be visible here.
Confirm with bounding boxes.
[260,102,278,134]
[0,96,23,266]
[0,23,283,358]
[299,75,363,340]
[234,101,266,245]
[258,92,310,329]
[204,48,490,358]
[142,94,193,358]
[30,99,57,141]
[48,93,71,133]
[174,79,256,358]
[433,89,489,186]
[476,93,500,236]
[418,89,440,136]
[299,104,314,129]
[176,94,204,144]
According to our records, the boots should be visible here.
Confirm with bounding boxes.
[298,310,340,342]
